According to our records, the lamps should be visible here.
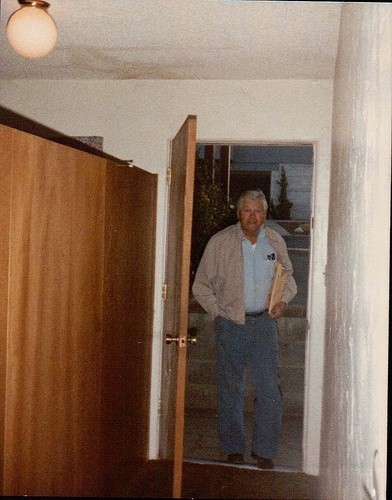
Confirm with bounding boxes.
[4,0,59,61]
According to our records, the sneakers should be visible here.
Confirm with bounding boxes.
[229,454,244,464]
[251,452,273,468]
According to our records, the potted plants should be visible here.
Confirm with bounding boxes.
[266,165,295,231]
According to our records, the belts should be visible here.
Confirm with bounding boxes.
[246,309,269,317]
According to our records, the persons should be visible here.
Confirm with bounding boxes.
[191,189,297,469]
